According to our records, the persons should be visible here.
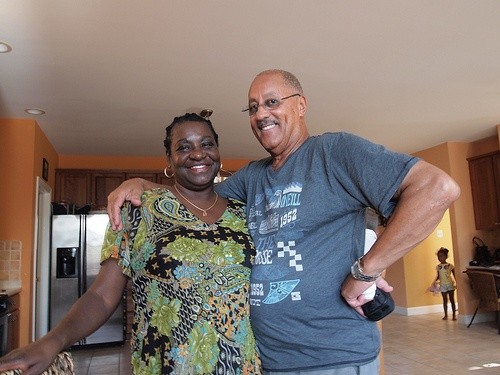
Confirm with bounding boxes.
[430,247,457,321]
[0,113,263,375]
[106,69,460,375]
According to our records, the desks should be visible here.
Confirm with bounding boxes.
[466,262,500,277]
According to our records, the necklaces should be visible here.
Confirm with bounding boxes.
[174,185,218,216]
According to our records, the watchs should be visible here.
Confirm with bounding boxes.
[350,256,382,282]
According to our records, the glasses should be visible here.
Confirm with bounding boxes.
[241,94,302,117]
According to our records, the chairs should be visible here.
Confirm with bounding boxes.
[467,271,500,334]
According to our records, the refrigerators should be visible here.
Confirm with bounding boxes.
[51,213,125,349]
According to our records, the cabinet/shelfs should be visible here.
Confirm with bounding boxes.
[54,168,93,206]
[0,292,20,359]
[91,169,175,209]
[467,150,500,230]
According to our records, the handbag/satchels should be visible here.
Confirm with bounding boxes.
[472,236,493,267]
